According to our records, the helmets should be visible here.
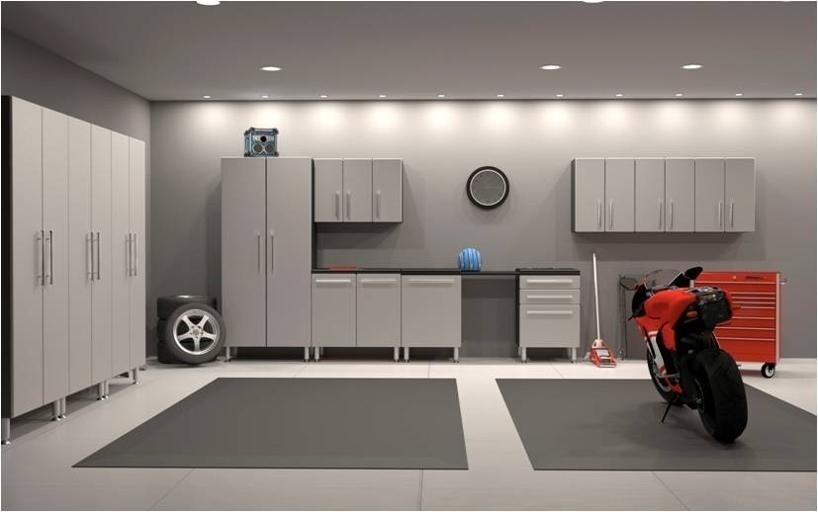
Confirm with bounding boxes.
[457,247,483,272]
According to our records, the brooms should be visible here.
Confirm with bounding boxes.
[582,251,610,363]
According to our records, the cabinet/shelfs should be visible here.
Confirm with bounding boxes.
[402,266,518,362]
[514,265,584,364]
[7,94,68,445]
[218,155,311,362]
[372,157,404,224]
[311,268,402,361]
[113,128,147,384]
[568,156,758,236]
[311,156,372,225]
[69,112,112,417]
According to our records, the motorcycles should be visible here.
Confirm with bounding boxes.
[615,264,752,448]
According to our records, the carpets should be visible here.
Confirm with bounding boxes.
[492,372,817,477]
[68,374,479,473]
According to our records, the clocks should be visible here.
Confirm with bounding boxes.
[465,164,510,210]
